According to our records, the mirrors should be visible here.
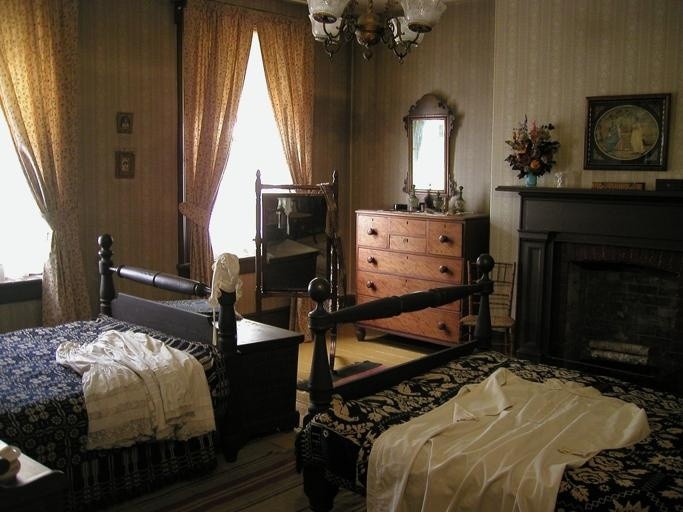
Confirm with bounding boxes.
[252,170,340,372]
[401,90,457,197]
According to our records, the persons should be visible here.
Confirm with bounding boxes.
[630,117,645,154]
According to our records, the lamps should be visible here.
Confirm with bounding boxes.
[305,0,447,67]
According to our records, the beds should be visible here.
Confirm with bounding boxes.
[301,255,682,512]
[0,228,239,505]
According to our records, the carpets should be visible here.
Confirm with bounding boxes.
[71,447,367,512]
[293,357,389,395]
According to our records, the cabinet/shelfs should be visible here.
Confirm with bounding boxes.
[352,209,488,346]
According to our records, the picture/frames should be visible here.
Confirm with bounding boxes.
[113,148,135,180]
[581,92,670,171]
[116,110,135,135]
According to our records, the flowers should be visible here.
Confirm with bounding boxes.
[505,112,561,178]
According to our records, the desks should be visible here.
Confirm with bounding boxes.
[199,309,304,439]
[265,236,322,290]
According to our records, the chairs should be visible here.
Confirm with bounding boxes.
[279,197,319,245]
[455,259,515,356]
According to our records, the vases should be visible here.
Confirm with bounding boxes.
[524,168,537,187]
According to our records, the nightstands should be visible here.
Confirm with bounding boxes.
[0,438,68,512]
